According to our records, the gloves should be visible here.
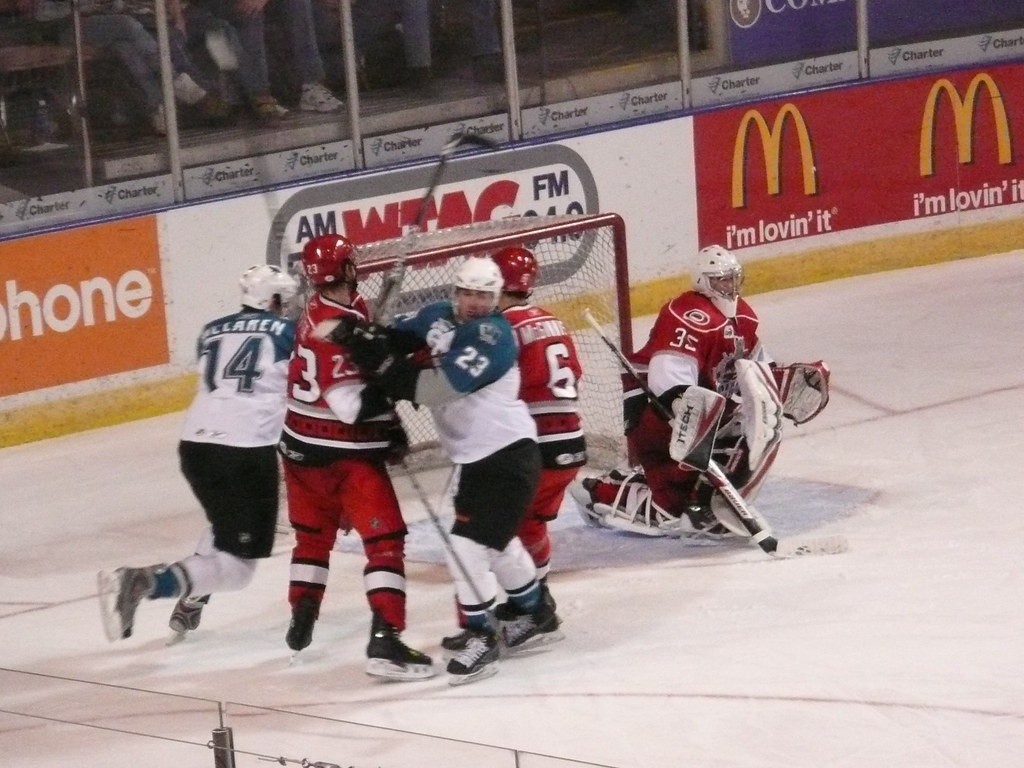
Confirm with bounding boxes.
[332,320,406,392]
[375,409,410,465]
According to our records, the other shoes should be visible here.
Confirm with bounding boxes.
[477,53,507,78]
[152,101,179,133]
[174,72,207,105]
[409,67,433,90]
[253,98,281,127]
[208,97,238,129]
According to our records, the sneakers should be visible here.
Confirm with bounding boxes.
[285,594,318,665]
[680,484,734,546]
[165,599,205,646]
[365,610,434,679]
[276,105,291,119]
[571,476,601,529]
[442,631,472,662]
[445,622,499,684]
[298,85,344,113]
[491,585,566,660]
[97,563,165,642]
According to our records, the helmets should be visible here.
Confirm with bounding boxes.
[301,234,356,289]
[454,257,504,314]
[690,244,744,318]
[240,264,298,313]
[489,248,538,294]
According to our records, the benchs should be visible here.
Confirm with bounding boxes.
[0,29,95,149]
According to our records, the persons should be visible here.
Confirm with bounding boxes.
[0,0,508,149]
[96,265,298,644]
[278,232,432,678]
[396,251,546,685]
[412,246,591,651]
[584,243,780,536]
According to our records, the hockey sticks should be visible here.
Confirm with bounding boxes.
[373,128,505,323]
[401,459,568,661]
[582,304,854,559]
